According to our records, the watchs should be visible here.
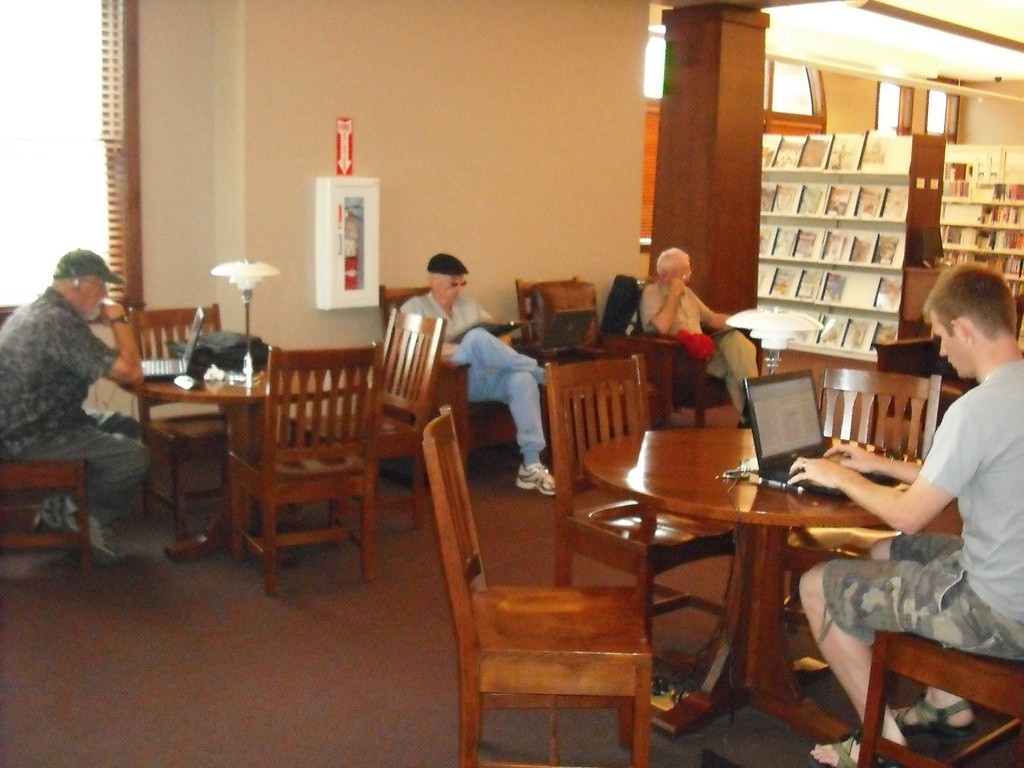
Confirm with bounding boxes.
[109,315,129,325]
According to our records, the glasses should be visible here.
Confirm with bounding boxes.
[681,270,693,280]
[447,281,469,289]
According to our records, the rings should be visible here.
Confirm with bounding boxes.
[802,462,806,472]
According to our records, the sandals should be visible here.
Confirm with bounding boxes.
[809,730,911,768]
[890,692,976,744]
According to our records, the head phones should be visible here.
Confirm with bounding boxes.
[67,251,79,288]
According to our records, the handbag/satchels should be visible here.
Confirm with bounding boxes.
[169,330,271,370]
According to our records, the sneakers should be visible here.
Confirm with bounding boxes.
[515,463,557,496]
[37,498,117,567]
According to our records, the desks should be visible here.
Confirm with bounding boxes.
[133,373,358,562]
[579,428,926,742]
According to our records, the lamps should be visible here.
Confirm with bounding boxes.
[211,260,281,387]
[725,308,824,376]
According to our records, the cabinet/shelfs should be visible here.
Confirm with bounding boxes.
[759,166,1024,438]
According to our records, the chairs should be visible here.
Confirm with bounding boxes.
[0,277,1024,767]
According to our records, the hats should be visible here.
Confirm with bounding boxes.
[427,254,469,276]
[53,249,125,285]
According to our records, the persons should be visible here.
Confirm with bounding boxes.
[639,247,759,429]
[401,255,556,496]
[0,248,150,564]
[787,264,1024,768]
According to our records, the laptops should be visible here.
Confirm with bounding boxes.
[744,368,898,496]
[518,307,597,352]
[141,305,205,379]
